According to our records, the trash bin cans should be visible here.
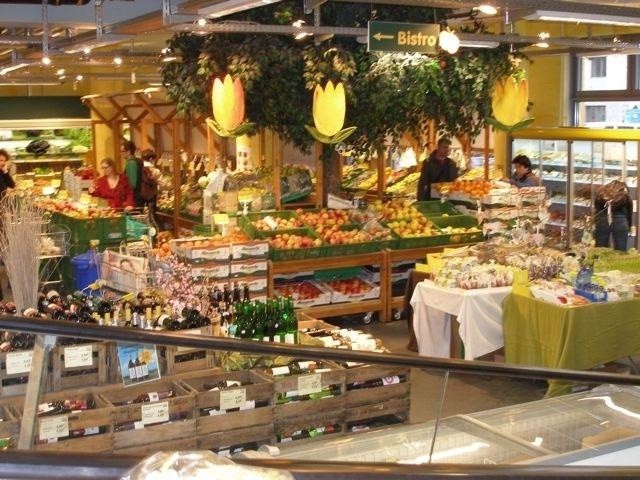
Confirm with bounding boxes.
[71,252,102,297]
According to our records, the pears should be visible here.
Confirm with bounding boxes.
[368,199,482,241]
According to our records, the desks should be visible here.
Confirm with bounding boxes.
[412,245,636,396]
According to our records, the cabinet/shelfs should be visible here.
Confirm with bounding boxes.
[493,127,636,248]
[267,243,466,326]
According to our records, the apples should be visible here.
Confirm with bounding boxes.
[252,208,392,250]
[278,277,373,302]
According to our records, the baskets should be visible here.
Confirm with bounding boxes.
[296,206,380,233]
[411,200,465,221]
[53,209,105,244]
[97,213,129,244]
[53,255,101,289]
[309,223,401,257]
[427,213,488,243]
[371,204,426,228]
[385,219,446,249]
[255,227,328,261]
[51,237,103,274]
[240,209,307,240]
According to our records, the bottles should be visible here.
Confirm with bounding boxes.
[575,263,640,304]
[154,151,235,183]
[1,280,408,461]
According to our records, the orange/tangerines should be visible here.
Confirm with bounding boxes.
[448,179,490,197]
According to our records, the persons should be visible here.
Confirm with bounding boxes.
[0,149,15,203]
[88,158,139,215]
[499,155,539,188]
[139,149,163,216]
[119,141,144,213]
[595,180,632,251]
[416,138,458,201]
